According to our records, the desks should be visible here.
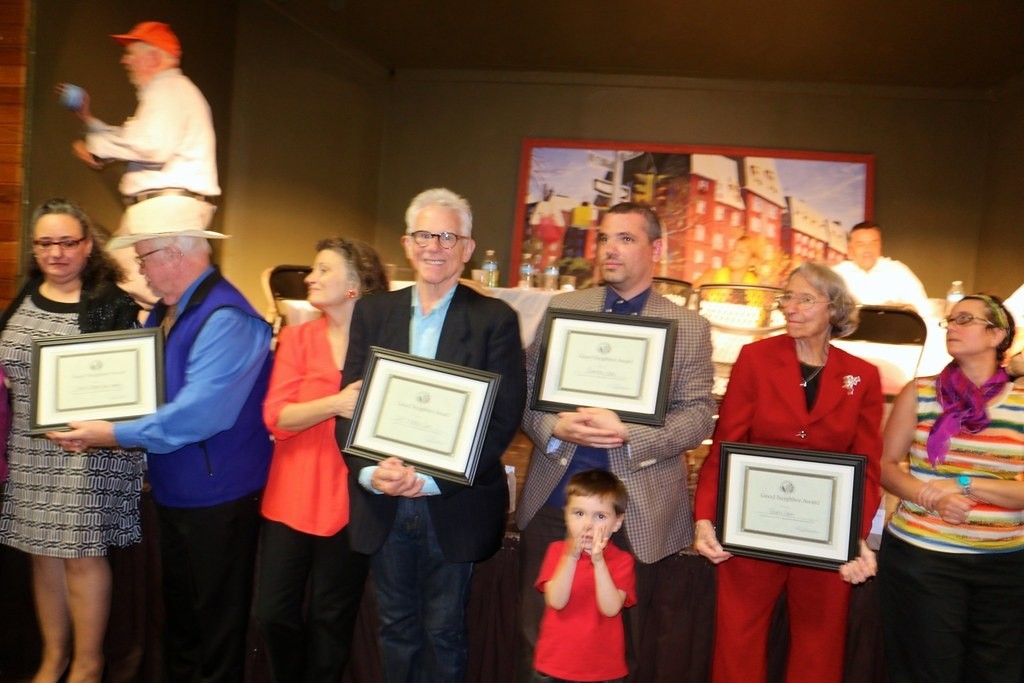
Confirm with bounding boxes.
[389,280,953,398]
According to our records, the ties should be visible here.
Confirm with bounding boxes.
[612,299,627,313]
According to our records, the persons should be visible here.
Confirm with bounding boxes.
[0,203,145,683]
[516,201,719,683]
[532,468,637,683]
[833,222,930,316]
[258,240,391,683]
[878,292,1023,683]
[335,189,527,683]
[692,264,885,683]
[56,23,221,324]
[45,195,274,683]
[698,236,764,304]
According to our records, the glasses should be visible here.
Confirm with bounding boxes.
[134,247,184,268]
[776,295,832,310]
[31,235,86,250]
[939,313,1000,329]
[408,230,469,249]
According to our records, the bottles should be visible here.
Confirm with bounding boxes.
[542,255,559,291]
[944,280,965,317]
[517,252,534,289]
[479,249,499,288]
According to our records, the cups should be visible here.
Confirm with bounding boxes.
[471,269,490,286]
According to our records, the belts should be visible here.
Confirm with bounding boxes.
[121,189,209,204]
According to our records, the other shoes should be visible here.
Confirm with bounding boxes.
[64,659,106,683]
[31,655,71,683]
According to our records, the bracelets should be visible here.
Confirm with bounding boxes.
[959,476,971,495]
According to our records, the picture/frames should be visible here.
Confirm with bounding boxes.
[342,345,501,487]
[715,442,868,573]
[530,307,679,426]
[27,325,166,436]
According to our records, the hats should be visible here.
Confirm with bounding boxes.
[104,196,231,251]
[110,22,183,59]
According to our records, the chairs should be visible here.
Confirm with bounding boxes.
[260,265,313,357]
[830,304,927,403]
[649,276,693,308]
[695,284,787,407]
